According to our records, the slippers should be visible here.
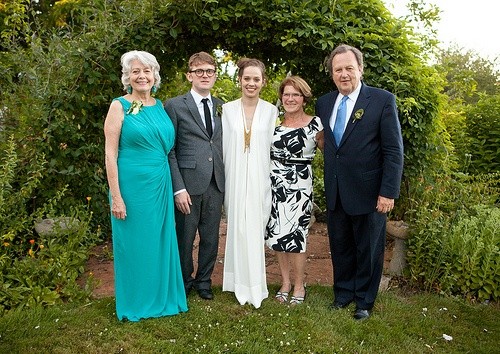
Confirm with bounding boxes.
[275,291,289,304]
[289,296,305,306]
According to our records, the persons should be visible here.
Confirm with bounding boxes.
[315,44,405,318]
[264,75,326,305]
[220,57,278,308]
[164,52,225,301]
[103,50,189,322]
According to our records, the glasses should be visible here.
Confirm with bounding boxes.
[191,69,216,77]
[282,93,303,98]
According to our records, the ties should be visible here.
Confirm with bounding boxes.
[201,99,214,138]
[333,95,349,148]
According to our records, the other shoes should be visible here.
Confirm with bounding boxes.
[329,300,348,312]
[198,289,213,299]
[353,308,369,319]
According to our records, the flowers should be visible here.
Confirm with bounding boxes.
[276,114,285,125]
[215,101,222,117]
[127,100,143,115]
[352,108,364,123]
